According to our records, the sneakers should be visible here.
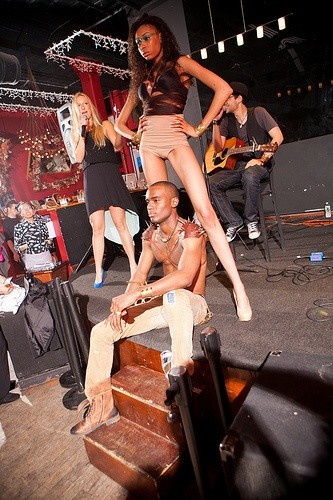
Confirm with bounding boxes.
[248,223,261,239]
[226,221,243,242]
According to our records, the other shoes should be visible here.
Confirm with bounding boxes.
[8,380,16,391]
[0,393,19,405]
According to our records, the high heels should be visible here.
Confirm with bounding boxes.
[93,269,104,288]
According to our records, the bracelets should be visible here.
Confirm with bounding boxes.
[212,118,221,126]
[259,155,270,164]
[139,285,154,300]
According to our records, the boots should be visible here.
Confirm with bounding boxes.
[70,377,120,436]
[160,350,195,423]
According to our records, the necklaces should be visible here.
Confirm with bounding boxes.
[157,215,180,243]
[236,111,247,128]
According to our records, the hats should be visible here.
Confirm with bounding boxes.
[229,82,248,100]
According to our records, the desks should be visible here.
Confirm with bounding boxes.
[15,261,68,282]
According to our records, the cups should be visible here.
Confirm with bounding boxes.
[77,193,84,203]
[126,174,136,189]
[60,199,67,206]
[138,172,148,189]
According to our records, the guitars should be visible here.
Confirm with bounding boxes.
[200,135,278,176]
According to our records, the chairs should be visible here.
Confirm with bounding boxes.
[227,171,286,263]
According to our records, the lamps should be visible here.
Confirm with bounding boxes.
[217,41,225,53]
[256,26,264,38]
[277,17,286,30]
[236,34,244,46]
[200,48,207,59]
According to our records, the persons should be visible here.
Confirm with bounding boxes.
[204,81,284,240]
[112,13,252,321]
[12,201,54,271]
[0,274,22,404]
[0,200,24,282]
[68,92,143,289]
[69,181,213,436]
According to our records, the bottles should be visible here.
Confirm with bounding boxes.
[325,202,331,218]
[25,270,35,288]
